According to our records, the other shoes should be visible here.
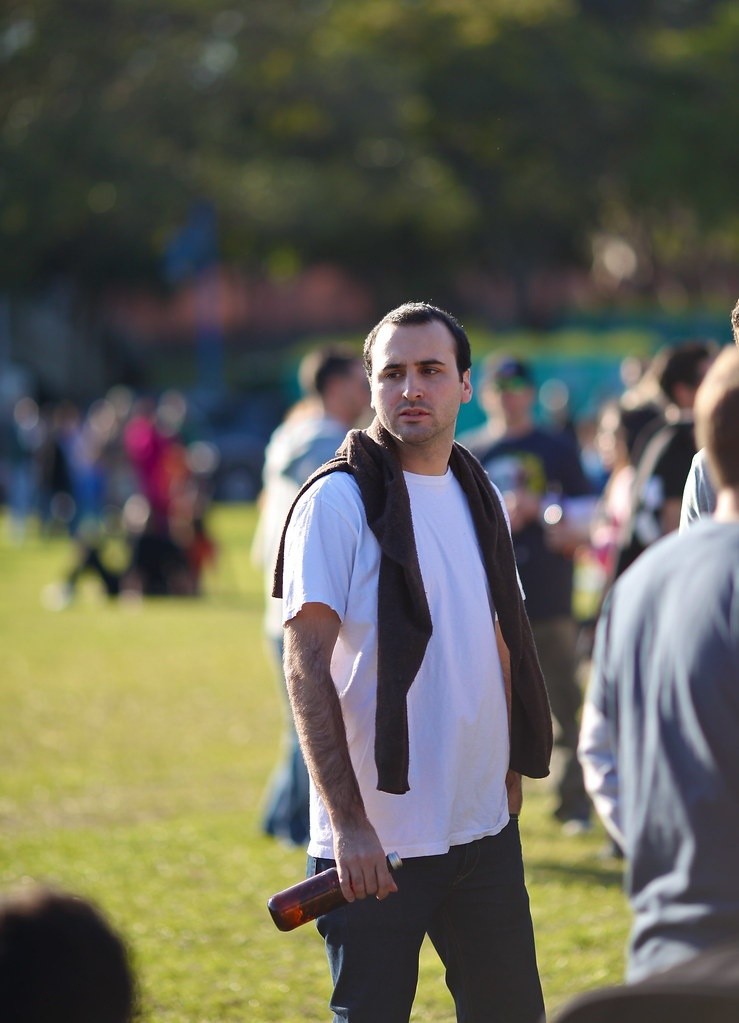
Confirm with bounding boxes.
[563,817,589,837]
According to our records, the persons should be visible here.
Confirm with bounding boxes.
[0,883,142,1023]
[251,351,370,847]
[572,301,738,984]
[455,358,597,836]
[284,301,553,1023]
[12,396,214,603]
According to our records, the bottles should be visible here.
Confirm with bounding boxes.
[269,850,401,931]
